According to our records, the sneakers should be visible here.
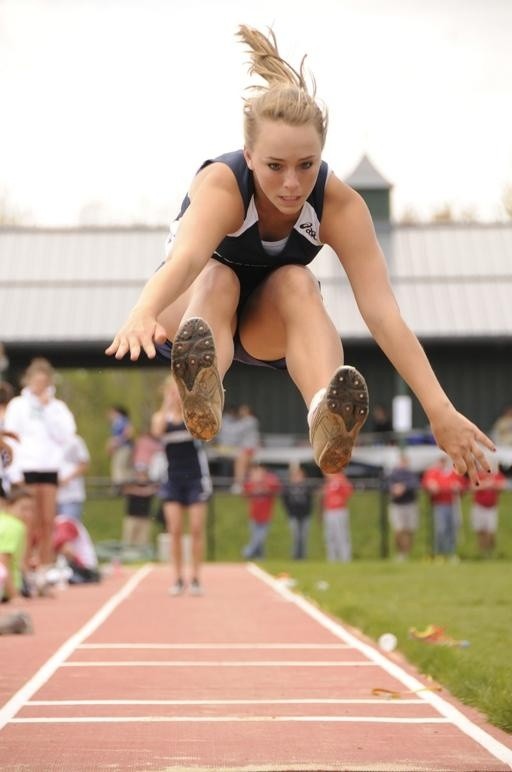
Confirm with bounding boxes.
[309,366,369,474]
[170,318,223,442]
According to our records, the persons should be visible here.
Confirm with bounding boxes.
[239,464,282,560]
[101,26,501,491]
[418,454,468,563]
[383,456,422,562]
[278,467,323,561]
[320,472,354,561]
[1,343,261,609]
[362,404,396,491]
[467,469,506,553]
